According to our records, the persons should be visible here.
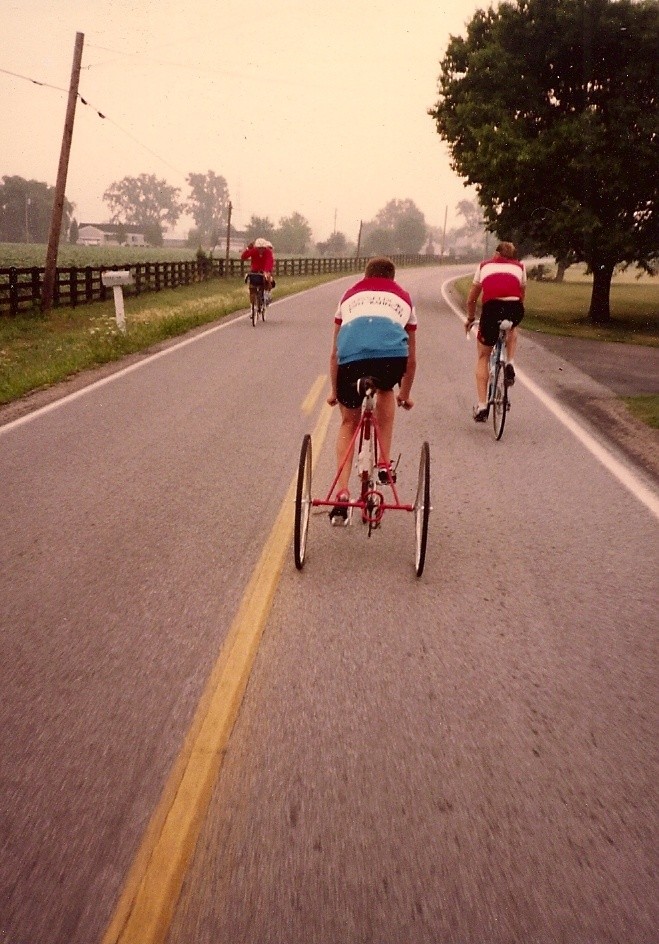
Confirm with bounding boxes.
[464,241,527,418]
[328,257,418,528]
[240,238,274,318]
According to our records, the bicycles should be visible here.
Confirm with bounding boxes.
[465,319,514,441]
[245,272,272,327]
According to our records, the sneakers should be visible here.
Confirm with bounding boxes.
[330,489,349,526]
[378,467,396,484]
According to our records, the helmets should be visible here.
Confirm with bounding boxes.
[255,238,265,248]
[265,241,272,248]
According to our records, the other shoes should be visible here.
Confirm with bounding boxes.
[473,403,487,421]
[504,362,515,387]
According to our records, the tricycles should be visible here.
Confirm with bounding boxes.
[292,374,434,577]
